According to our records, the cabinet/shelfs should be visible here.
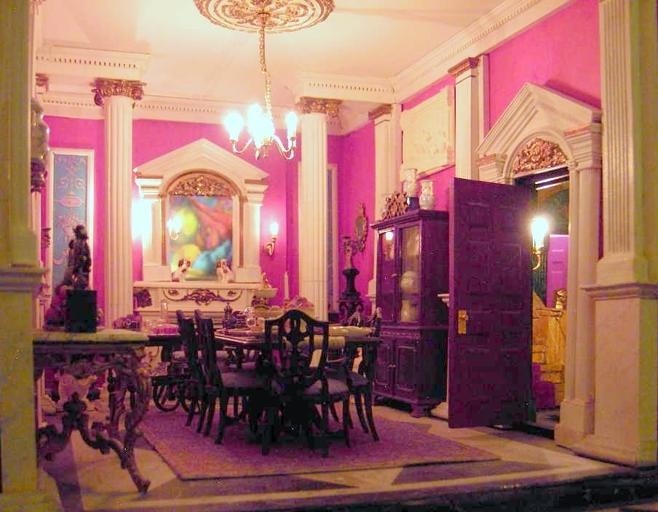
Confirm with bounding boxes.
[368,210,448,419]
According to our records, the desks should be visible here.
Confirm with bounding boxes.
[33,325,152,500]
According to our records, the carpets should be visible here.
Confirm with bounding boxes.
[136,400,509,484]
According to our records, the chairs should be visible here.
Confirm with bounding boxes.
[149,309,384,461]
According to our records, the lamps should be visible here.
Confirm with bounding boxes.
[189,0,336,162]
[530,213,553,274]
[165,213,186,242]
[262,221,283,259]
